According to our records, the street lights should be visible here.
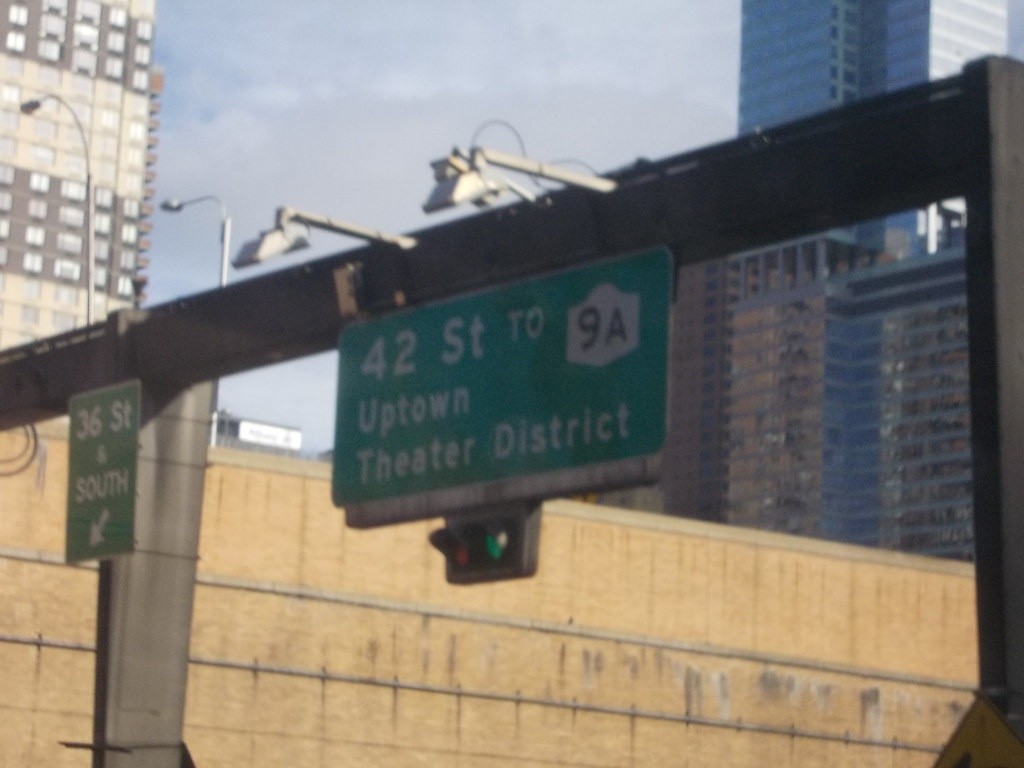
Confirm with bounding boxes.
[20,94,94,338]
[159,191,234,446]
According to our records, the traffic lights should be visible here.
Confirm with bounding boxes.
[429,497,544,587]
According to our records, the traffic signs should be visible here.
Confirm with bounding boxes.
[64,379,142,563]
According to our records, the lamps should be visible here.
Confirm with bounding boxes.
[226,204,420,277]
[421,145,618,224]
